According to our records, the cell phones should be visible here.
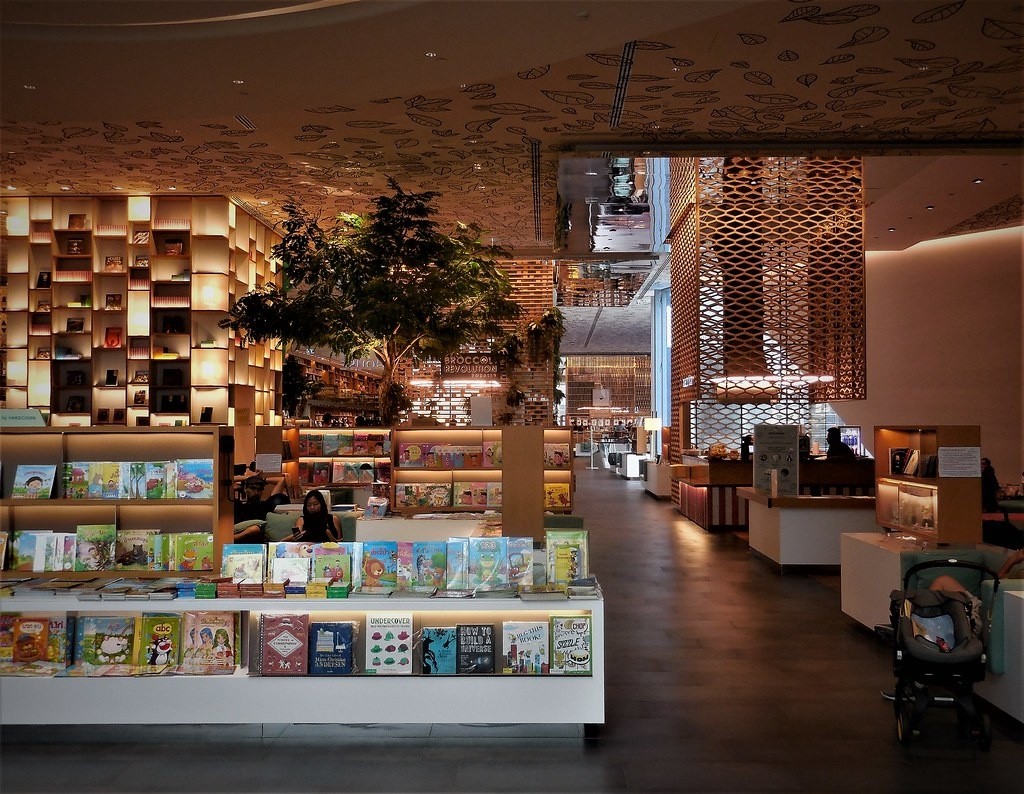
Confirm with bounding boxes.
[292,527,302,538]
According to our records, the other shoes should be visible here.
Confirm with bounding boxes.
[874,624,894,640]
[881,683,930,701]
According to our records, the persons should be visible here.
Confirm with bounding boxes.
[825,427,855,458]
[291,489,343,542]
[981,458,1002,513]
[282,405,289,419]
[571,422,632,431]
[234,470,284,544]
[299,412,368,427]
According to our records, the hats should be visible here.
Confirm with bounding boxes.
[323,414,334,420]
[244,476,263,484]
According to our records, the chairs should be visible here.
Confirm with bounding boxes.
[888,588,983,667]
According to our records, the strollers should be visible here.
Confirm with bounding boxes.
[889,555,1001,756]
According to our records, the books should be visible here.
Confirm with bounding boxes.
[0,525,604,600]
[889,446,936,478]
[544,484,570,507]
[395,481,502,525]
[298,433,391,520]
[0,214,216,426]
[12,459,213,500]
[399,441,502,467]
[544,443,570,470]
[0,610,592,677]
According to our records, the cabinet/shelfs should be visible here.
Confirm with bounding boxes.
[1,190,646,748]
[871,423,984,544]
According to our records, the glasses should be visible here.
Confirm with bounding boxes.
[248,485,264,491]
